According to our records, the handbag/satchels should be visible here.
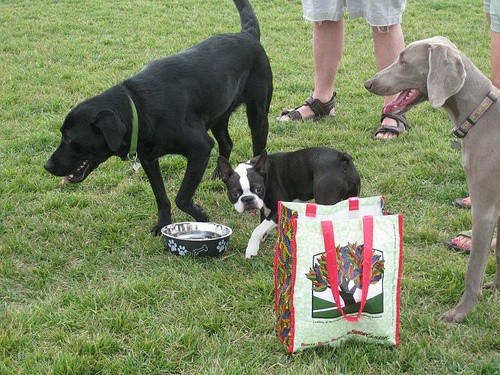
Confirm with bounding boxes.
[273,200,401,354]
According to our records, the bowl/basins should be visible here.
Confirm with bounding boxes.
[160,221,232,258]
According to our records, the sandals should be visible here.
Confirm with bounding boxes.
[373,107,408,138]
[455,196,471,209]
[450,227,499,252]
[279,91,338,122]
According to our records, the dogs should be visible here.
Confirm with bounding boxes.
[364,35,500,324]
[42,0,275,238]
[217,147,363,260]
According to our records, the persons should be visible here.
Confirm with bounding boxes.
[275,0,412,141]
[444,0,500,254]
